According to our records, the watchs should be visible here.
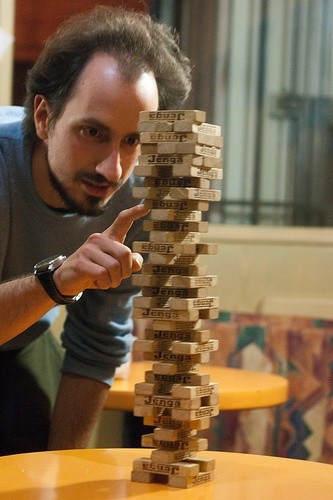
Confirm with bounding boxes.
[34,255,83,305]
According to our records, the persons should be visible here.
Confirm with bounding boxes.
[0,5,191,456]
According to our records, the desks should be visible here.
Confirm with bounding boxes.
[0,450,333,500]
[105,360,288,450]
